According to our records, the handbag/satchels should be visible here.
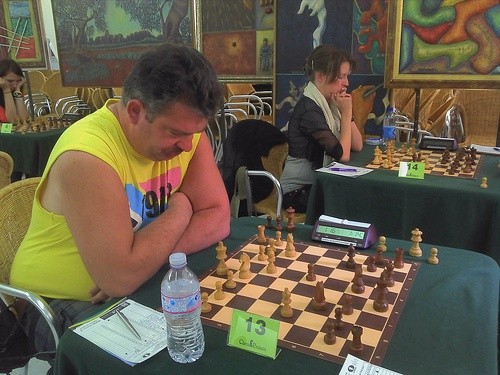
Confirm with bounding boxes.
[230,165,250,220]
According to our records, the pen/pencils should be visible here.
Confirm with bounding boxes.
[331,168,357,172]
[115,309,142,341]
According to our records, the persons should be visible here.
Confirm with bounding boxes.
[276,45,364,213]
[0,57,28,122]
[8,41,231,351]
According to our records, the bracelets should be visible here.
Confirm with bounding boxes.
[351,116,354,122]
[3,92,11,94]
[12,90,23,100]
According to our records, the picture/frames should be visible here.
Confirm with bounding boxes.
[0,1,49,71]
[192,0,273,84]
[383,0,500,88]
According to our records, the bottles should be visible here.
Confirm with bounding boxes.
[383,108,396,147]
[160,252,205,364]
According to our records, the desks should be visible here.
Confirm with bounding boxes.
[58,217,499,375]
[315,141,500,266]
[0,129,67,178]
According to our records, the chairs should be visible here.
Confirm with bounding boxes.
[0,68,435,351]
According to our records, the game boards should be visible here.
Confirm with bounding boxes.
[366,146,484,180]
[196,233,420,366]
[12,121,57,133]
